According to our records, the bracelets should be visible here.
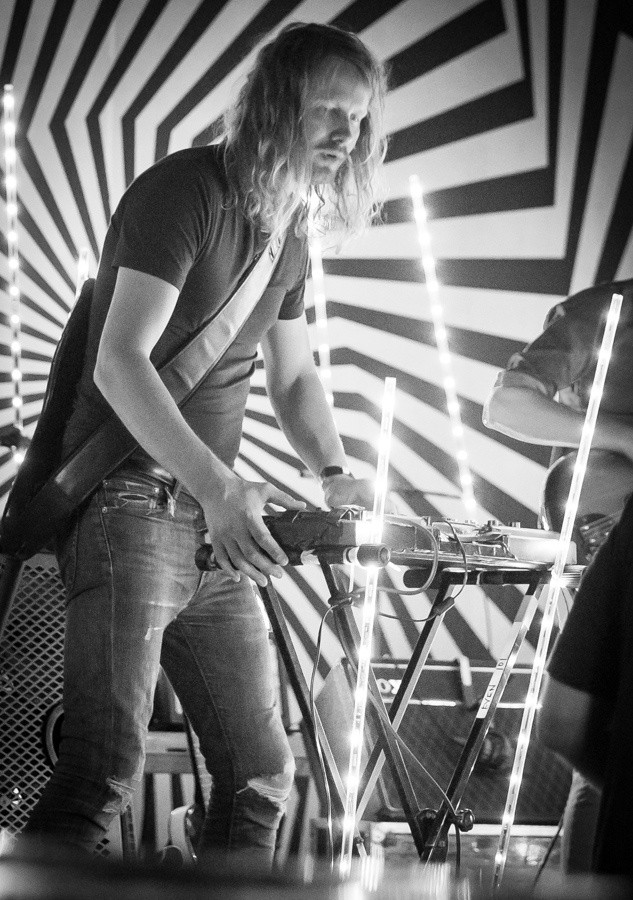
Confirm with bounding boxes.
[319,465,355,484]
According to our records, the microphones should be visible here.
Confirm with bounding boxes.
[453,729,513,769]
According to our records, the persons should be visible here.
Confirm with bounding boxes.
[14,22,396,874]
[482,277,633,870]
[535,495,633,900]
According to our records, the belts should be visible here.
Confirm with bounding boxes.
[129,448,194,498]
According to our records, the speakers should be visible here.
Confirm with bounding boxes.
[342,657,575,824]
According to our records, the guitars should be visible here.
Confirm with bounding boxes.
[545,446,633,570]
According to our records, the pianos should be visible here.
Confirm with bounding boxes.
[198,508,576,874]
